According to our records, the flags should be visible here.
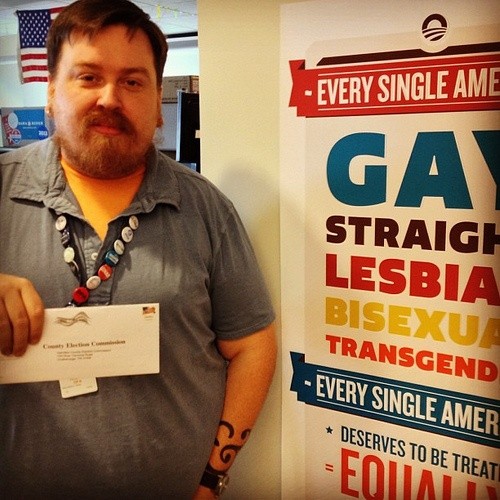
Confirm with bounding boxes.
[16,6,65,84]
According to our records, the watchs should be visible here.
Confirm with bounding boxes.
[199,471,230,495]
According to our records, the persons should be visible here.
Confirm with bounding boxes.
[1,2,277,499]
